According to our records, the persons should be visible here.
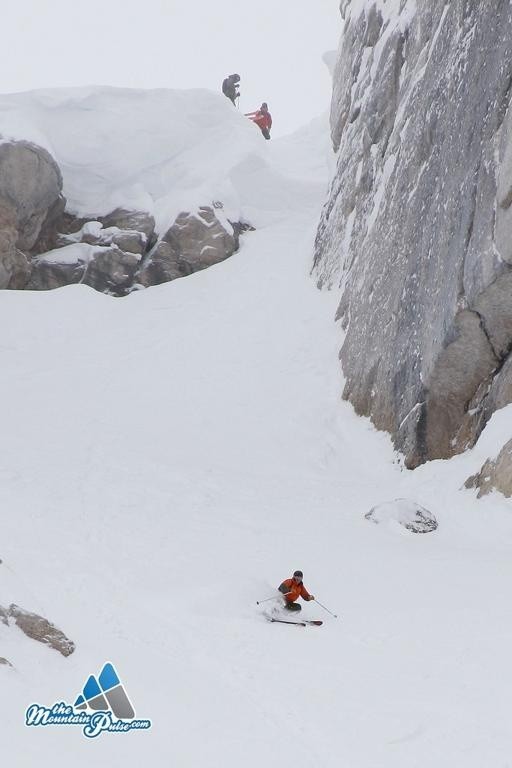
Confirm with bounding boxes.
[221,73,241,107]
[277,570,315,612]
[244,102,272,140]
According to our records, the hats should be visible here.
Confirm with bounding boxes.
[294,571,303,577]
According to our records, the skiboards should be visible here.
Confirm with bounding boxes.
[269,618,323,626]
